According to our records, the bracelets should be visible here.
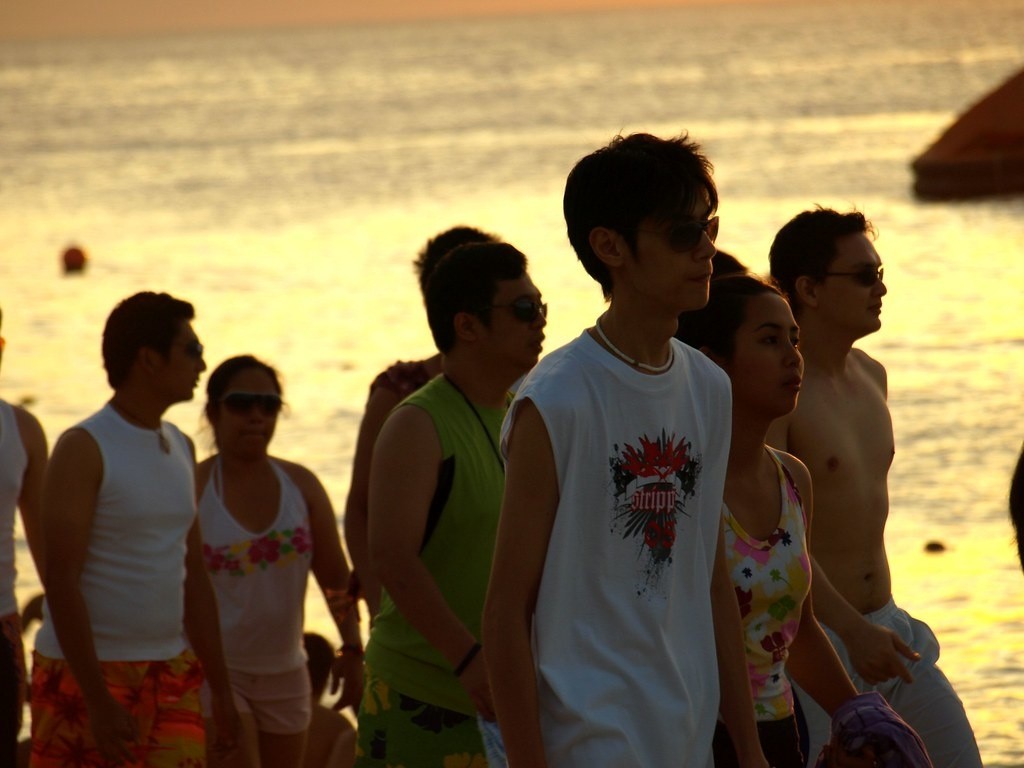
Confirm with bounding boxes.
[453,643,483,679]
[341,644,367,662]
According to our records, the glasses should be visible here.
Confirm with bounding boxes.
[493,302,549,323]
[218,391,280,414]
[817,267,884,287]
[637,216,719,252]
[164,340,203,359]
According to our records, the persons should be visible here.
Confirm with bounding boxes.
[0,132,1024,768]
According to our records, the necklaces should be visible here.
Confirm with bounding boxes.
[595,312,674,372]
[443,373,506,476]
